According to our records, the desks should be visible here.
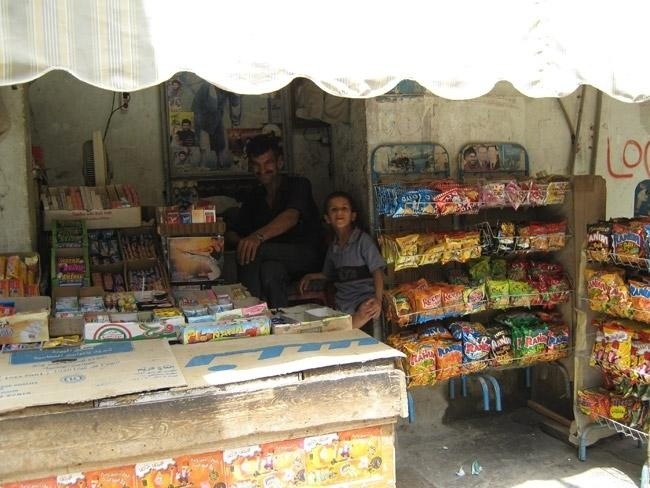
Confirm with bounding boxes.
[171,283,271,345]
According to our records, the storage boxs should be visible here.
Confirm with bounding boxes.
[49,287,174,337]
[267,302,352,333]
[37,192,228,295]
[83,309,185,344]
[1,252,50,345]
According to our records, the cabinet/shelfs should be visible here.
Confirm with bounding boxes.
[371,141,577,423]
[577,178,650,488]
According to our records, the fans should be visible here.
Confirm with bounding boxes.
[81,129,114,187]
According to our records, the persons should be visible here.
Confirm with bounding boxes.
[226,133,328,298]
[287,191,386,332]
[464,145,500,170]
[169,75,243,173]
